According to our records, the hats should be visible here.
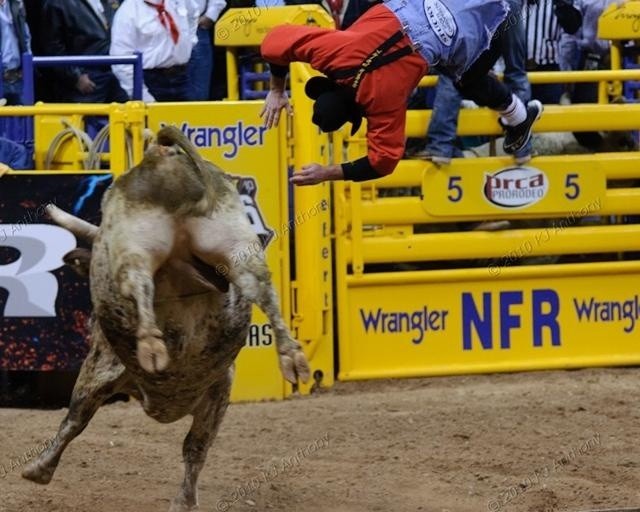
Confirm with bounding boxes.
[304,76,363,136]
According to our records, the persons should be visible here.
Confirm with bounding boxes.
[1,1,639,186]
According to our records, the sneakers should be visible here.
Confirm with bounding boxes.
[414,147,465,164]
[498,100,542,164]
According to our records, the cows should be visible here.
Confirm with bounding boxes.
[19,125,312,511]
